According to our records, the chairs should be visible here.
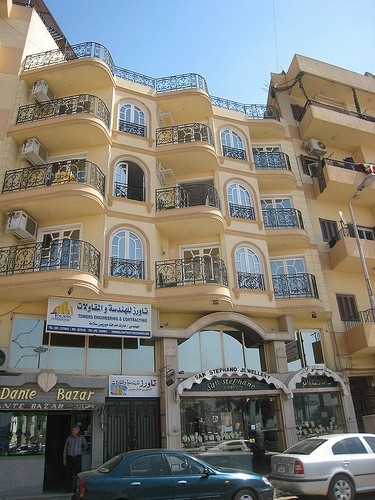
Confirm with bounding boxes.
[159,273,177,288]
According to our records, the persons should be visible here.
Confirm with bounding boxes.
[223,413,230,426]
[63,425,88,494]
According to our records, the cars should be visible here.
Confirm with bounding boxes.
[72,448,275,500]
[207,439,271,454]
[270,433,375,500]
[9,443,36,455]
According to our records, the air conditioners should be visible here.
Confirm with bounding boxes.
[32,80,56,104]
[5,210,37,241]
[21,137,48,166]
[306,138,327,156]
